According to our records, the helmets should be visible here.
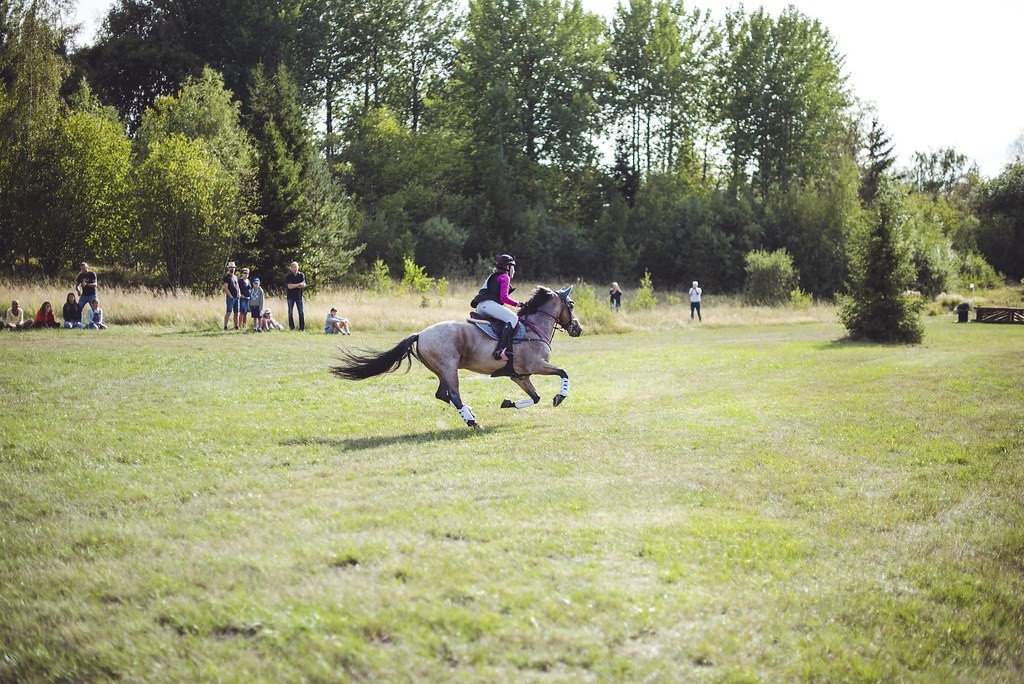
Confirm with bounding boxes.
[496,254,516,266]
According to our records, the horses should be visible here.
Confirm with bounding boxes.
[330,285,584,432]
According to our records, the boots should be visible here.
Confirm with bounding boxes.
[495,322,513,360]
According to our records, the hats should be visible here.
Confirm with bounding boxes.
[253,278,260,286]
[265,309,271,314]
[227,262,236,268]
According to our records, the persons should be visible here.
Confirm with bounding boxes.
[0,263,108,330]
[224,261,353,336]
[609,282,622,312]
[470,255,526,362]
[689,281,702,323]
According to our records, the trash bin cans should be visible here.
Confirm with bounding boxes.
[957,303,970,323]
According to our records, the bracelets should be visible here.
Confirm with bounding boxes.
[87,283,88,285]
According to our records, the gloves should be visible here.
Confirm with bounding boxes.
[518,302,528,307]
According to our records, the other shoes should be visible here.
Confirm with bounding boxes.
[348,333,351,335]
[253,328,261,333]
[343,333,346,335]
